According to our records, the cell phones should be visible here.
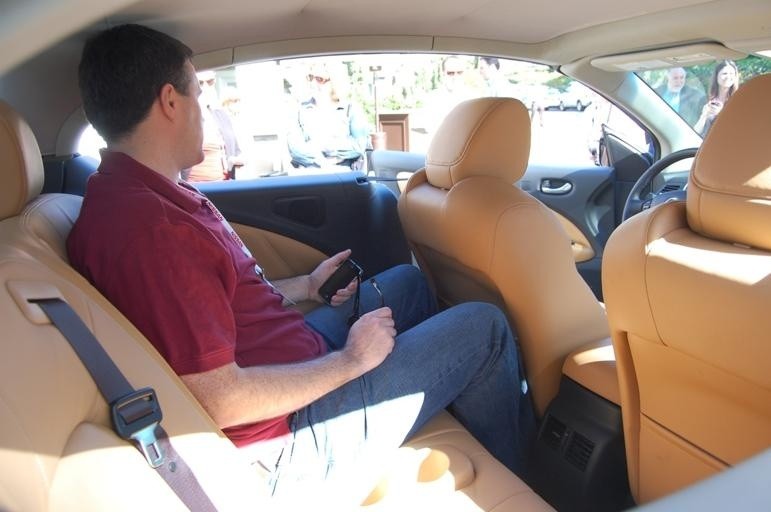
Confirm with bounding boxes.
[318,259,364,305]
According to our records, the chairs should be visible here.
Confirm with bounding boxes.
[599,72,771,505]
[396,97,611,419]
[0,100,558,512]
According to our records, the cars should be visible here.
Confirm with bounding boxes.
[520,87,651,167]
[185,92,376,183]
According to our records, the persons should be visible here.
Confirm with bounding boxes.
[65,24,537,470]
[178,56,650,183]
[645,67,702,156]
[691,60,740,140]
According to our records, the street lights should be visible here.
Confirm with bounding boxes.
[368,64,385,86]
